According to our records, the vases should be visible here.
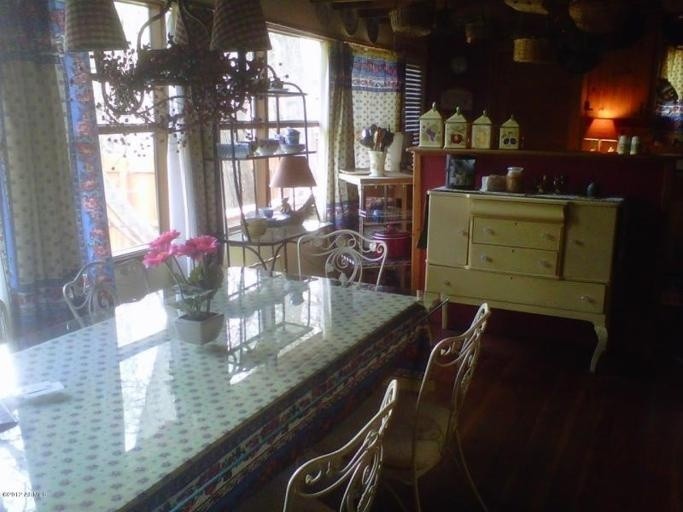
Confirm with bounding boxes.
[174,309,225,345]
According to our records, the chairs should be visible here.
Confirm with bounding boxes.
[296,230,387,287]
[229,379,400,511]
[61,260,121,328]
[327,301,491,512]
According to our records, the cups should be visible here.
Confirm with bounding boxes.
[368,150,386,177]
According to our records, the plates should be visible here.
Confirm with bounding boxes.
[216,142,249,161]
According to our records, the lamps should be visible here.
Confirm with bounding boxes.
[268,156,317,231]
[209,1,272,52]
[64,1,129,54]
[172,2,210,53]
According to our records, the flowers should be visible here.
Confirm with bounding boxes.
[142,229,223,316]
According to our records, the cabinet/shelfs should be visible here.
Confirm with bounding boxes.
[406,144,680,296]
[423,183,620,374]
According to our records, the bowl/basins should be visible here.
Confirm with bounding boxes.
[257,139,279,157]
[242,219,266,239]
[281,144,305,155]
[262,209,274,218]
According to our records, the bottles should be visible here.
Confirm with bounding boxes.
[505,167,524,193]
